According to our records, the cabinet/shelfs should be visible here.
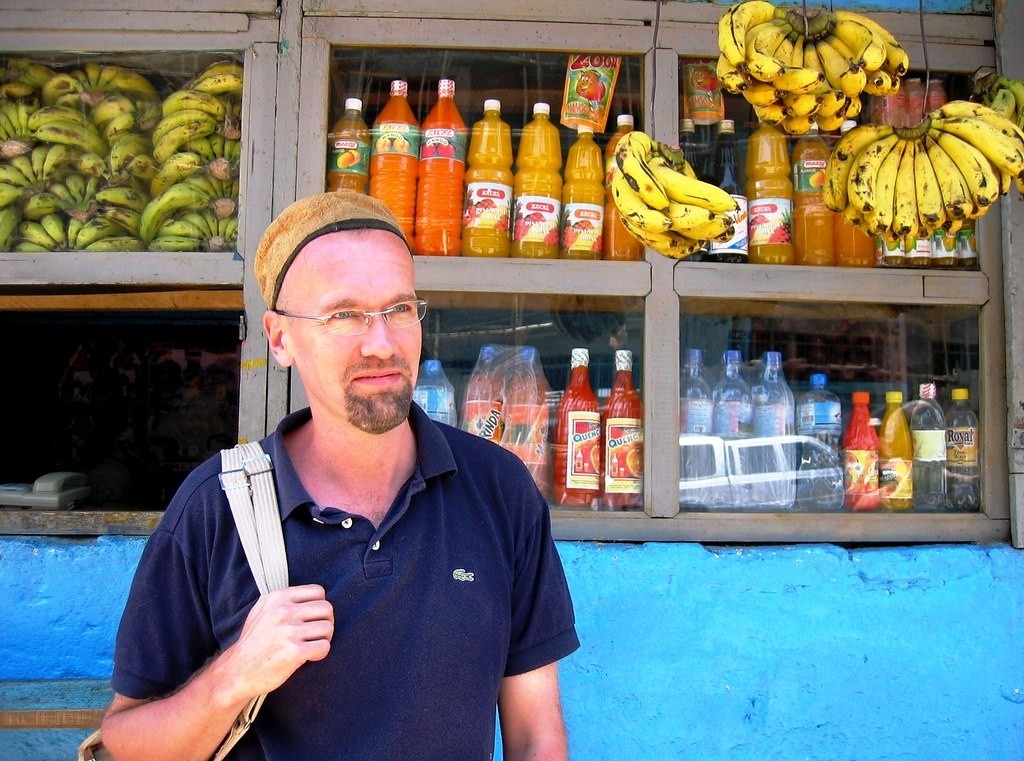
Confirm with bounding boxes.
[0,0,1012,548]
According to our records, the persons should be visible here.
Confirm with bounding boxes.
[101,189,580,761]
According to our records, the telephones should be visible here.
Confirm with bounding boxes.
[0,472,96,509]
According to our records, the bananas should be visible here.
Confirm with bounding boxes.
[822,71,1024,242]
[610,131,738,258]
[0,53,244,253]
[716,0,909,134]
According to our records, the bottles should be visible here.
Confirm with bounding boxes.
[461,345,549,506]
[370,80,420,254]
[412,360,458,430]
[875,219,976,267]
[713,351,753,512]
[328,98,371,194]
[561,123,605,260]
[462,99,514,258]
[792,122,837,266]
[511,102,563,258]
[680,349,713,510]
[604,115,645,261]
[745,118,793,265]
[601,350,644,510]
[911,384,980,513]
[555,348,600,511]
[679,119,701,262]
[796,374,844,511]
[869,77,946,129]
[833,121,875,268]
[844,392,879,511]
[414,79,468,257]
[753,352,796,512]
[879,391,914,511]
[701,120,749,262]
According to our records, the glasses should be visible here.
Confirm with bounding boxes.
[273,297,427,336]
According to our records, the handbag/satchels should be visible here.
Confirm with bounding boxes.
[79,440,288,761]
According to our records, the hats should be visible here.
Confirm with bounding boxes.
[254,191,414,313]
